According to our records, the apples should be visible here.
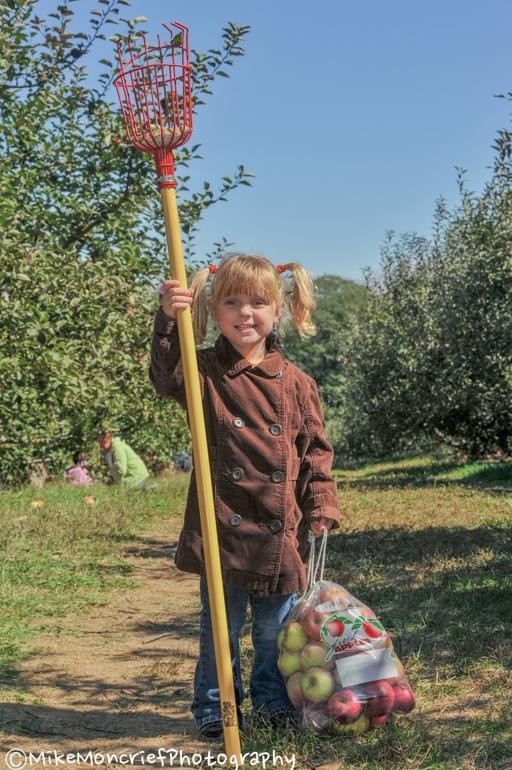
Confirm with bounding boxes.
[278,585,414,736]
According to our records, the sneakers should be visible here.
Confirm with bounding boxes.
[254,708,301,732]
[195,705,247,742]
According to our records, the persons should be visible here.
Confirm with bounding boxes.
[148,249,346,741]
[92,430,150,490]
[64,451,95,484]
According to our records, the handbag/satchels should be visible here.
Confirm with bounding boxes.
[274,520,422,738]
